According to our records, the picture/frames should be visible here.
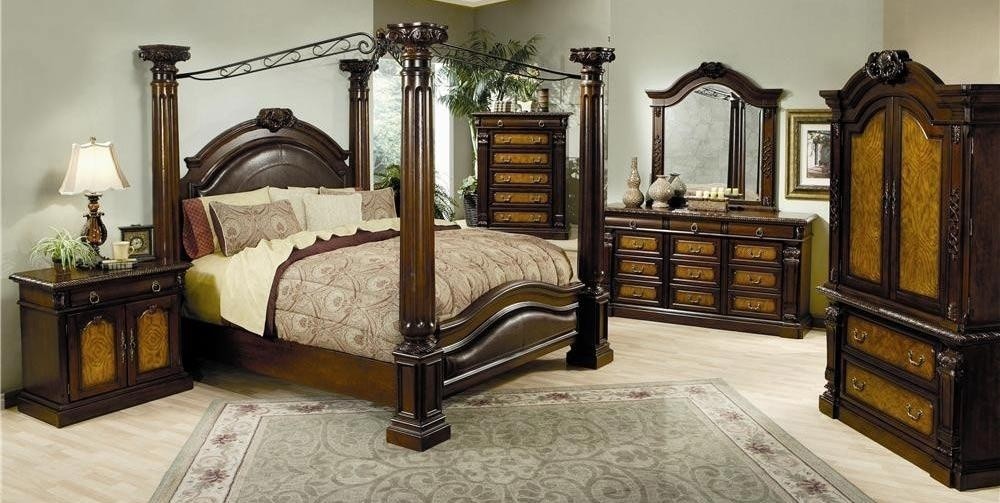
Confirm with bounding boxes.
[784,109,832,200]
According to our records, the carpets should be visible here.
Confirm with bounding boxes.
[146,377,879,503]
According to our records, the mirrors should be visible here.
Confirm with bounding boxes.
[645,61,783,213]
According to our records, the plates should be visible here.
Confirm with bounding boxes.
[103,259,137,266]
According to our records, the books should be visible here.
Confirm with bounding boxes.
[102,262,138,270]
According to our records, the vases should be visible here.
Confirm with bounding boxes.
[648,175,675,208]
[623,157,644,211]
[668,173,687,198]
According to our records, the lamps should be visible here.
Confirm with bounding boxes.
[57,136,131,260]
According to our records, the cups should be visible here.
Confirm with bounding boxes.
[113,240,134,259]
[695,190,724,198]
[711,187,739,195]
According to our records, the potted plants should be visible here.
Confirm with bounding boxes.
[431,25,521,227]
[29,224,99,273]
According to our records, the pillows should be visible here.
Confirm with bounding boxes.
[182,185,398,260]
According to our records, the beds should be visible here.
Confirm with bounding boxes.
[138,21,616,452]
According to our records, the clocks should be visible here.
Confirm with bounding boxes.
[118,224,156,257]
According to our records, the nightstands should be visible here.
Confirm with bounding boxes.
[9,258,193,428]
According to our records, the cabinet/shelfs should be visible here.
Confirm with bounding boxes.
[471,112,574,240]
[604,203,819,339]
[816,51,1000,491]
